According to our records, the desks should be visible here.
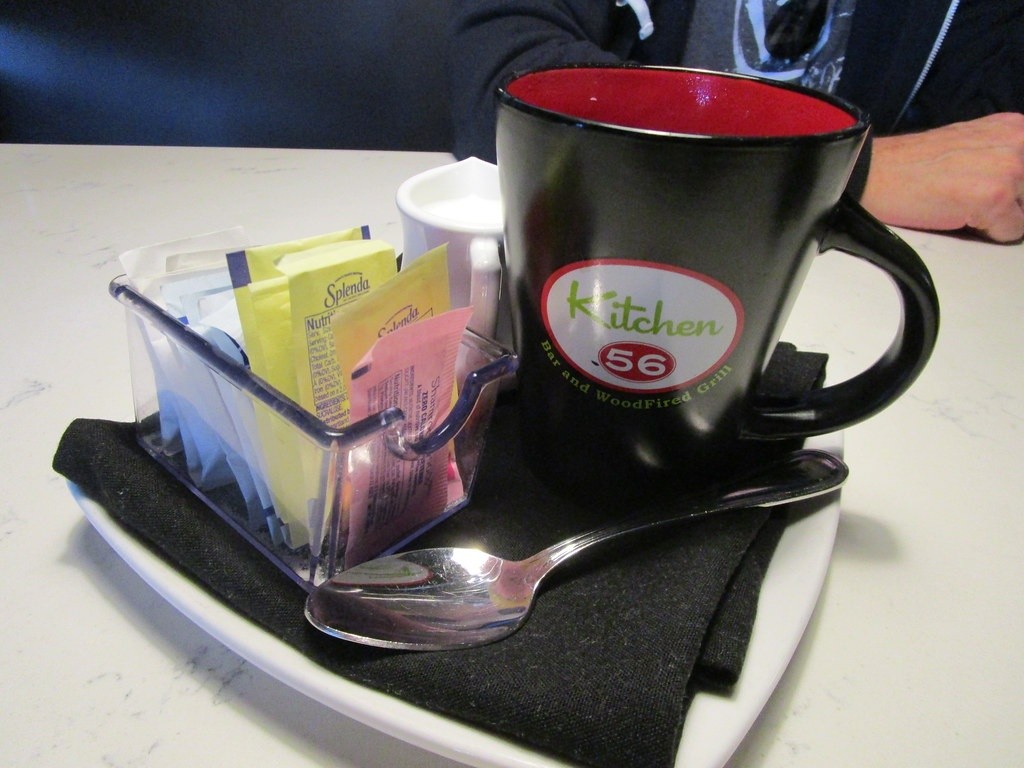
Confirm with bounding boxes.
[0,141,1024,768]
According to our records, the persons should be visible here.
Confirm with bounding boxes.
[443,0,1024,242]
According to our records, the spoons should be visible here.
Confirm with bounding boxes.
[305,449,849,650]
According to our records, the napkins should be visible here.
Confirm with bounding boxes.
[53,341,829,768]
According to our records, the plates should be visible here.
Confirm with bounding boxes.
[66,429,844,768]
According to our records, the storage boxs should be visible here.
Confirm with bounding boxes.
[109,273,518,591]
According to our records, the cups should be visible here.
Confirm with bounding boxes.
[494,63,940,467]
[398,156,517,384]
